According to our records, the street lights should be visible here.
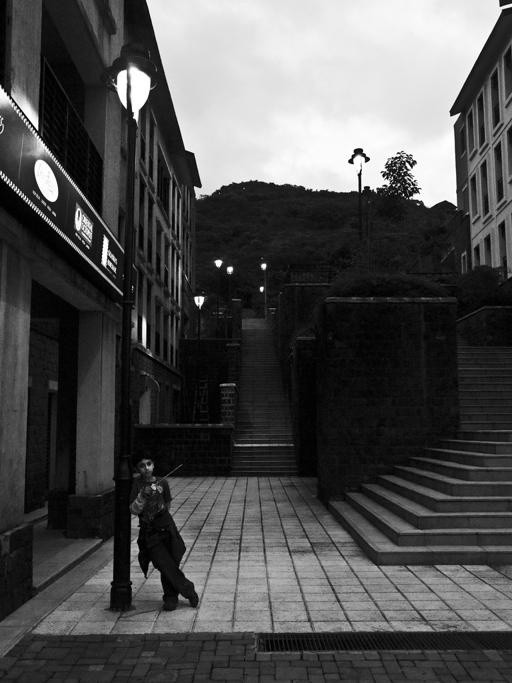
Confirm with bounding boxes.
[347,148,371,242]
[98,41,158,614]
[226,265,234,297]
[259,262,271,317]
[214,259,223,322]
[192,295,210,423]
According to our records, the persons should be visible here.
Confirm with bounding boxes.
[128,450,200,613]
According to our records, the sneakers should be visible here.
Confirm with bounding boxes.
[163,597,179,611]
[185,581,199,608]
